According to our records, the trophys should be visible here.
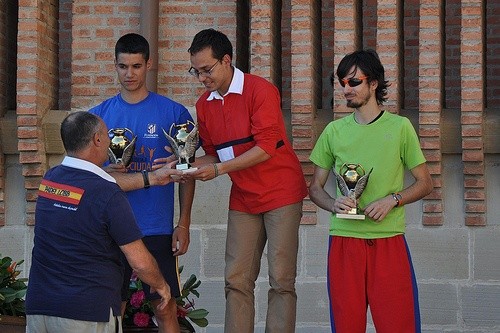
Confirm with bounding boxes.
[331,162,374,220]
[162,120,200,175]
[107,128,138,166]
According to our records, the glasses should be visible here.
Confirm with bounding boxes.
[337,76,364,87]
[189,60,220,77]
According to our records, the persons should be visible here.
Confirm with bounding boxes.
[84,33,198,333]
[26,111,171,333]
[307,50,433,333]
[150,29,308,333]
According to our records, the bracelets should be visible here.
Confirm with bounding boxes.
[178,225,192,233]
[396,192,406,207]
[212,163,219,178]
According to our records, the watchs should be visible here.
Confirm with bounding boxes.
[385,192,399,208]
[141,169,150,187]
